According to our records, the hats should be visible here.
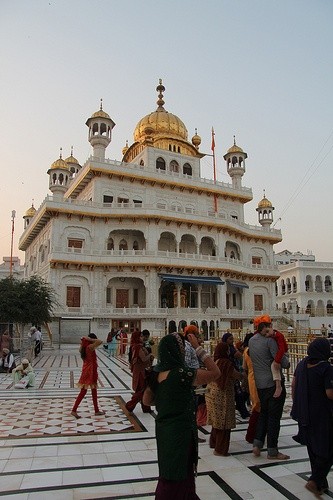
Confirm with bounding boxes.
[22,359,29,364]
[3,348,10,354]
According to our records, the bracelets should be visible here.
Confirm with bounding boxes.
[195,346,211,363]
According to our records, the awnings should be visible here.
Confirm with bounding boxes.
[157,272,225,286]
[226,280,249,288]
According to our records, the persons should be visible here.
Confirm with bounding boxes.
[0,330,17,373]
[105,316,333,497]
[161,296,167,308]
[70,333,106,419]
[26,324,44,357]
[12,358,36,389]
[141,333,222,500]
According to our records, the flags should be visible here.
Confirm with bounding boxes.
[211,131,216,150]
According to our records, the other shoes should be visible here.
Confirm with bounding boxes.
[71,412,81,418]
[95,412,104,415]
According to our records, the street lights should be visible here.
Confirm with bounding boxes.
[8,209,16,354]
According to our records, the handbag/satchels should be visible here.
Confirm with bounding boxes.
[15,376,30,388]
